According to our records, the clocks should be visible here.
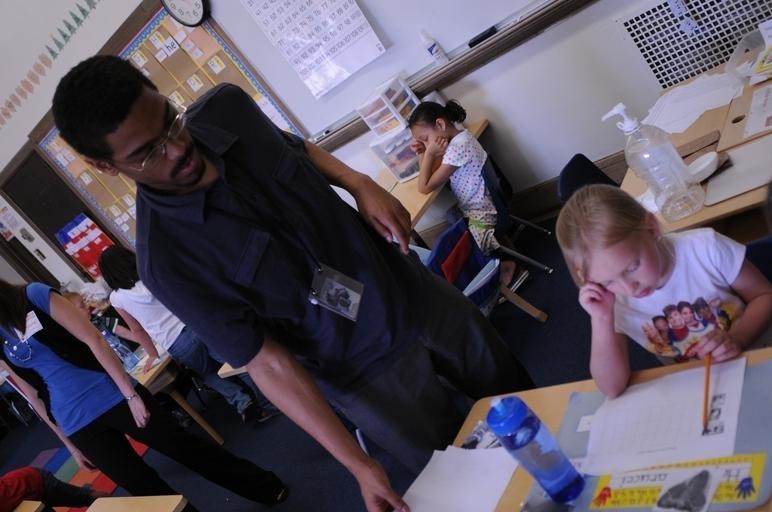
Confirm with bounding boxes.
[159,0,208,27]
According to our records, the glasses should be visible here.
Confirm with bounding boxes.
[127,97,187,171]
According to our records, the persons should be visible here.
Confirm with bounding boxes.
[0,279,288,512]
[52,55,537,512]
[0,467,114,512]
[61,292,139,366]
[98,245,284,422]
[556,184,772,399]
[406,100,516,286]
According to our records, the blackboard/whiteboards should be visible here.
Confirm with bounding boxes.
[206,0,597,153]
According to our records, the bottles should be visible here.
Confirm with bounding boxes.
[102,330,140,370]
[416,29,450,67]
[486,396,585,502]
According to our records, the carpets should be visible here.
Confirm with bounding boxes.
[27,434,150,512]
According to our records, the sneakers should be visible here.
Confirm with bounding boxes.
[498,265,529,303]
[242,401,280,423]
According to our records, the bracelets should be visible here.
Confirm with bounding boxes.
[126,393,138,402]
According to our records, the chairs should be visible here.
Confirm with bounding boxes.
[556,152,622,205]
[427,155,555,323]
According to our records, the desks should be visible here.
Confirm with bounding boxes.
[120,343,224,446]
[447,346,771,512]
[84,494,186,511]
[12,499,44,511]
[361,119,515,249]
[620,43,771,246]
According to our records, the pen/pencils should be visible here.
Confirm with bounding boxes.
[312,130,330,144]
[703,352,710,430]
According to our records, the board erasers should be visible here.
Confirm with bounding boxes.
[468,25,497,49]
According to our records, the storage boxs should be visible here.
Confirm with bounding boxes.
[355,75,464,184]
